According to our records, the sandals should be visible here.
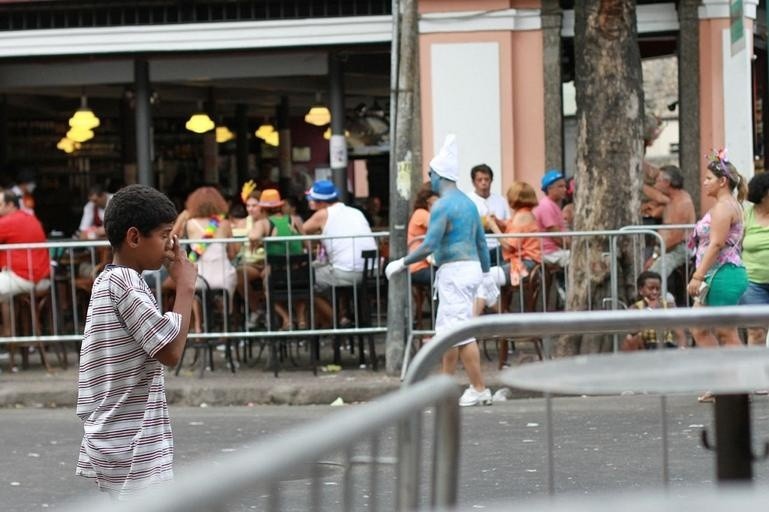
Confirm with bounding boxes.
[698,391,715,402]
[278,322,308,344]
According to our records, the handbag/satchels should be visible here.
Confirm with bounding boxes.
[687,281,710,305]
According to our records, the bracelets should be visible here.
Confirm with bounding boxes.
[692,274,704,281]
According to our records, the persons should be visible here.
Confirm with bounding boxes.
[618,162,696,351]
[686,161,750,403]
[408,163,574,313]
[385,135,493,407]
[75,182,200,496]
[73,181,379,331]
[741,172,768,395]
[1,188,51,337]
[6,166,39,215]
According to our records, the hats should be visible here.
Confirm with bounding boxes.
[305,179,339,202]
[430,155,459,181]
[567,179,574,195]
[540,169,563,190]
[255,189,285,209]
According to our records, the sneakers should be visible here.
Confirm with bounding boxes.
[245,310,263,330]
[216,337,250,353]
[0,346,35,361]
[331,318,351,347]
[459,384,492,406]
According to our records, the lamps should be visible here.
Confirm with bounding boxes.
[57,93,99,153]
[305,91,332,127]
[211,111,236,143]
[255,116,280,147]
[323,124,350,141]
[186,97,216,133]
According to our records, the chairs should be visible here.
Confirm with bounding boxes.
[165,232,387,379]
[406,227,569,370]
[0,232,113,373]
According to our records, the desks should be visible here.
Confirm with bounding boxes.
[504,346,769,485]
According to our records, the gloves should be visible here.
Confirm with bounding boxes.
[480,272,500,307]
[385,257,407,280]
